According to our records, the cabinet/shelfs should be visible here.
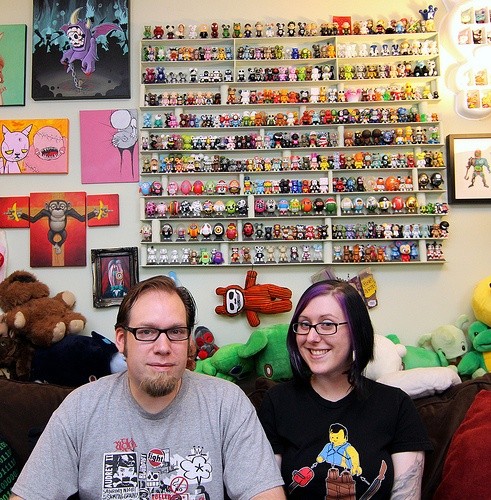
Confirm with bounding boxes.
[139,33,449,268]
[441,1,491,122]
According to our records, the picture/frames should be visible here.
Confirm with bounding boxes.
[91,247,139,309]
[445,133,491,205]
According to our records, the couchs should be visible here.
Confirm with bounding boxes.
[0,377,490,500]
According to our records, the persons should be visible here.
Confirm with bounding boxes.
[258,279,431,500]
[306,22,339,36]
[255,21,263,37]
[341,22,350,35]
[287,21,296,36]
[10,275,286,500]
[244,24,252,38]
[160,92,213,106]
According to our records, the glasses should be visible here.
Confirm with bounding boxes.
[292,322,349,335]
[119,323,193,342]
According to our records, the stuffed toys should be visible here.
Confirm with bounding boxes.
[0,270,129,387]
[361,276,491,395]
[193,324,293,383]
[215,271,292,327]
